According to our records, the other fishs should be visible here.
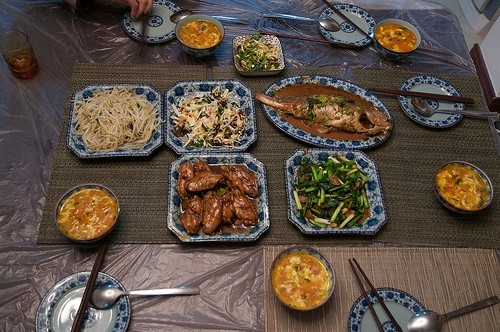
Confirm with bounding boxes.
[255,91,393,134]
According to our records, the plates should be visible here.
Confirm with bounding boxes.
[122,0,181,45]
[399,76,464,127]
[263,75,392,149]
[318,5,375,50]
[283,149,388,236]
[35,271,130,332]
[68,79,271,243]
[348,287,426,332]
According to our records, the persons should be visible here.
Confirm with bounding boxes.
[65,0,153,21]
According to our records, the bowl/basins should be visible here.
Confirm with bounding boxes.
[373,18,421,61]
[434,160,494,216]
[233,34,285,77]
[54,182,120,242]
[271,246,336,312]
[176,15,225,56]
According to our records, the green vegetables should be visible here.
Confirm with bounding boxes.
[189,97,225,148]
[305,94,356,125]
[293,157,370,228]
[236,34,280,71]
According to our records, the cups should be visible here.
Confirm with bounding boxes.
[0,31,40,79]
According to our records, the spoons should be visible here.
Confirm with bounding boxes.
[261,11,341,31]
[406,296,500,332]
[410,98,499,119]
[91,286,199,309]
[169,10,249,25]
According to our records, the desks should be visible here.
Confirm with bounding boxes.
[0,0,500,331]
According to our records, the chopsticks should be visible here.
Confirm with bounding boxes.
[347,258,403,332]
[261,0,373,49]
[368,88,474,104]
[71,241,109,332]
[138,13,148,58]
